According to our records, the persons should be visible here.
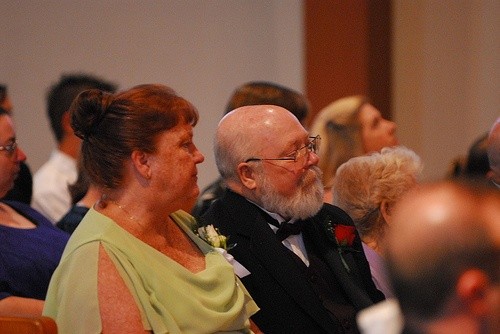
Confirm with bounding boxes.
[0,71,500,334]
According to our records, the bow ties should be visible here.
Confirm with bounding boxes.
[247,201,302,242]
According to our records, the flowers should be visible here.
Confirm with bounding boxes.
[328,222,363,272]
[194,224,237,251]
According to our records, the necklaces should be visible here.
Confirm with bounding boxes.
[108,198,149,232]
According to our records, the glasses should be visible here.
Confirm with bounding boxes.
[0,140,19,157]
[245,134,322,163]
